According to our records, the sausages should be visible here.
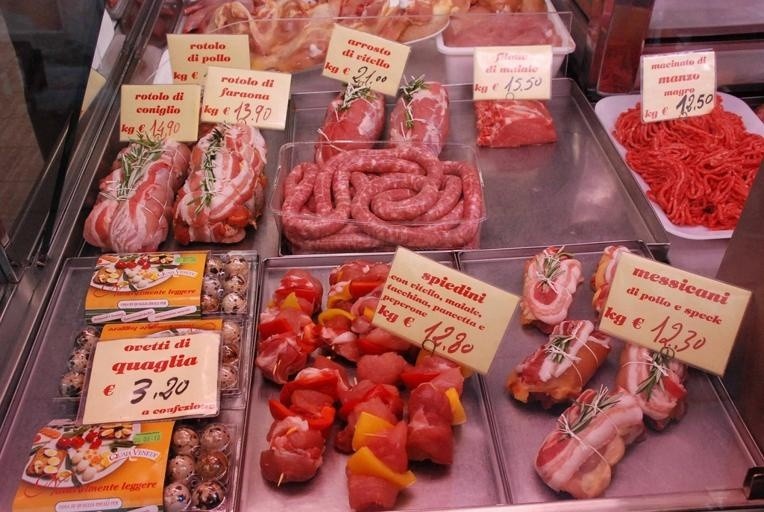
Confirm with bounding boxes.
[280,143,484,251]
